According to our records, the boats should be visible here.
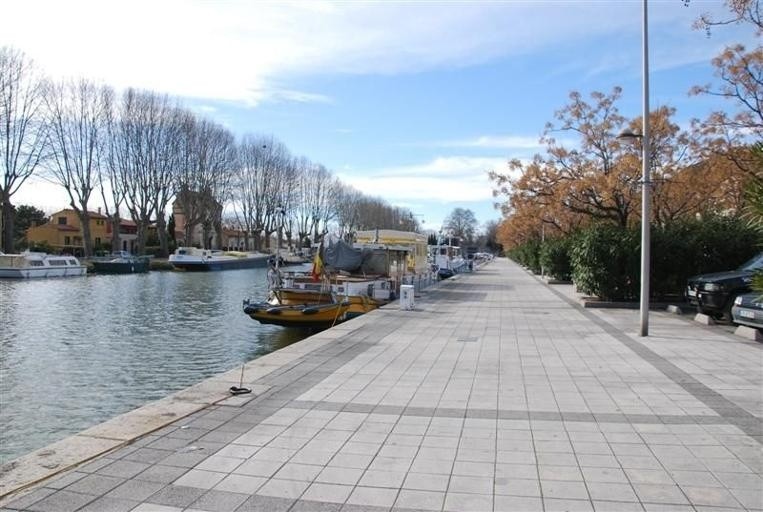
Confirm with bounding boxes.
[425,228,468,278]
[92,255,150,274]
[167,246,269,272]
[243,229,441,328]
[271,248,305,267]
[1,248,90,279]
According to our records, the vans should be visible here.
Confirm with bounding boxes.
[111,250,131,257]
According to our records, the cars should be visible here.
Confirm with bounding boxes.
[730,290,763,330]
[683,252,762,321]
